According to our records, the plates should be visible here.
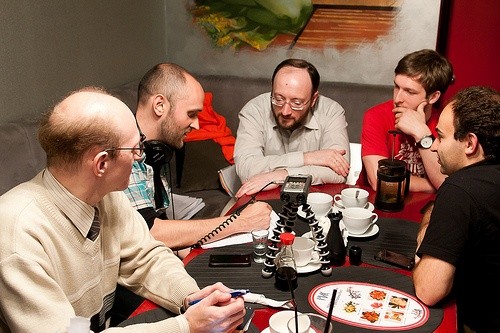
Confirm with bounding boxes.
[296,203,340,220]
[296,261,322,274]
[333,199,377,214]
[343,223,380,238]
[261,326,316,333]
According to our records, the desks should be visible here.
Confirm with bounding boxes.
[131,183,458,333]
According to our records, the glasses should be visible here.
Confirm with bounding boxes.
[270,94,312,111]
[104,134,147,157]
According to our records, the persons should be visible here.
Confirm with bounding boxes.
[0,84,246,333]
[354,49,449,192]
[233,58,351,199]
[110,63,272,327]
[412,84,500,333]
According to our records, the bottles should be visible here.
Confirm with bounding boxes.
[325,213,346,267]
[274,233,298,292]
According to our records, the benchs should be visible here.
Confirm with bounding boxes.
[0,74,395,219]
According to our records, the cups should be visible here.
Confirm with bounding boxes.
[306,192,334,217]
[334,188,370,210]
[269,310,332,333]
[252,228,270,263]
[342,207,378,234]
[293,237,316,267]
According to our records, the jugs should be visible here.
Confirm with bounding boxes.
[374,158,411,212]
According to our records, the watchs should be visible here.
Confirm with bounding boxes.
[416,133,436,150]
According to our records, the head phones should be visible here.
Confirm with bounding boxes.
[134,117,172,168]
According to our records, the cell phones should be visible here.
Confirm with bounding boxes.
[374,248,416,271]
[209,254,251,267]
[187,288,250,306]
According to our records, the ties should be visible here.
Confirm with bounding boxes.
[86,206,100,241]
